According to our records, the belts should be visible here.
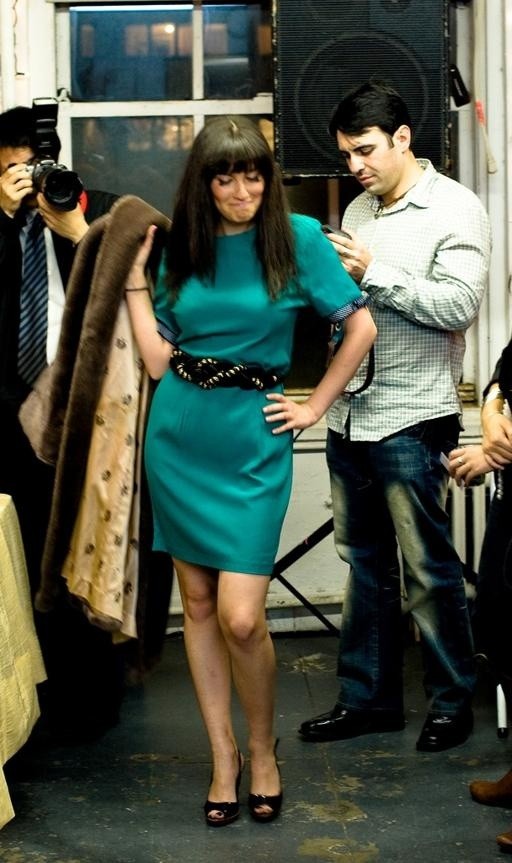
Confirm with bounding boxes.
[168,345,285,391]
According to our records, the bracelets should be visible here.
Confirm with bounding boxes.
[124,287,148,291]
[481,387,505,410]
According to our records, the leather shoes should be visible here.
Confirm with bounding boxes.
[204,749,243,827]
[467,767,512,809]
[415,704,476,754]
[298,701,408,742]
[495,830,511,853]
[248,738,284,824]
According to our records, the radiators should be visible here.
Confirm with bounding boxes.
[398,472,496,642]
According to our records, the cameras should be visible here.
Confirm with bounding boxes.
[8,97,83,211]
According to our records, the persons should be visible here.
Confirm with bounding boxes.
[446,336,512,858]
[121,115,378,825]
[294,86,492,748]
[1,104,174,743]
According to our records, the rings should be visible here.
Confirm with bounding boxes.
[457,455,464,464]
[342,247,351,257]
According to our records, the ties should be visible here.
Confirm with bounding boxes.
[16,210,51,387]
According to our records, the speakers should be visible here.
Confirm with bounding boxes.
[272,0,451,179]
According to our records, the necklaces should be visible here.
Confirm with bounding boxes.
[374,183,415,218]
